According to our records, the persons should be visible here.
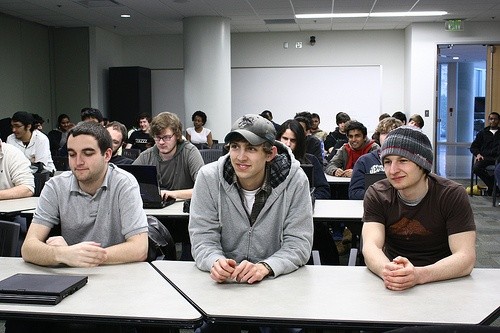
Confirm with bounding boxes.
[131,111,205,261]
[81,107,156,166]
[259,110,425,266]
[469,112,500,206]
[185,111,213,149]
[0,109,76,178]
[21,120,150,267]
[0,138,35,258]
[188,114,313,284]
[360,125,477,291]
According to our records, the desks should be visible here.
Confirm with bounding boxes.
[313,197,364,222]
[151,253,500,333]
[0,249,204,333]
[0,196,40,218]
[20,191,190,220]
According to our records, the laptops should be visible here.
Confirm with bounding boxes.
[116,164,176,209]
[0,272,88,305]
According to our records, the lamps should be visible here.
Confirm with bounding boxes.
[443,18,464,32]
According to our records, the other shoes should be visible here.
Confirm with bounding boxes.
[487,187,493,196]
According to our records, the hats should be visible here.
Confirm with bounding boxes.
[380,125,432,172]
[224,113,275,147]
[409,114,424,129]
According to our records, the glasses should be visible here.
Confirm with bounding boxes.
[153,133,174,141]
[11,125,23,129]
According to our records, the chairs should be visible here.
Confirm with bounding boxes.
[470,129,495,197]
[0,220,21,257]
